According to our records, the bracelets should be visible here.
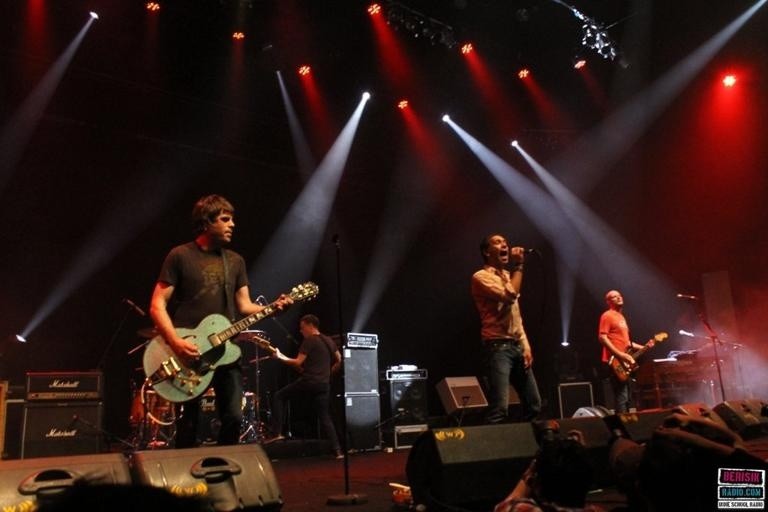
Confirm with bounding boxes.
[509,262,525,272]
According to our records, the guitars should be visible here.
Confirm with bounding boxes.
[142,283,319,402]
[609,332,668,381]
[254,338,305,374]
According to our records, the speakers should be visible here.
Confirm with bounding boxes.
[345,394,382,450]
[711,400,768,440]
[533,416,613,473]
[672,402,730,431]
[20,400,103,459]
[603,409,680,443]
[379,379,430,424]
[406,422,541,512]
[0,453,131,512]
[556,382,594,419]
[343,350,378,393]
[133,444,285,512]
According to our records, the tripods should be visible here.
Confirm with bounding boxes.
[239,346,278,443]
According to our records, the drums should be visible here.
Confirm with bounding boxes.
[127,390,174,430]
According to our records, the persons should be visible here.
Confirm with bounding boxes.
[465,231,546,430]
[267,313,349,463]
[137,191,297,455]
[595,283,658,411]
[493,411,768,512]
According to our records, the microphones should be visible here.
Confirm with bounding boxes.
[523,248,534,253]
[675,293,695,302]
[122,298,148,320]
